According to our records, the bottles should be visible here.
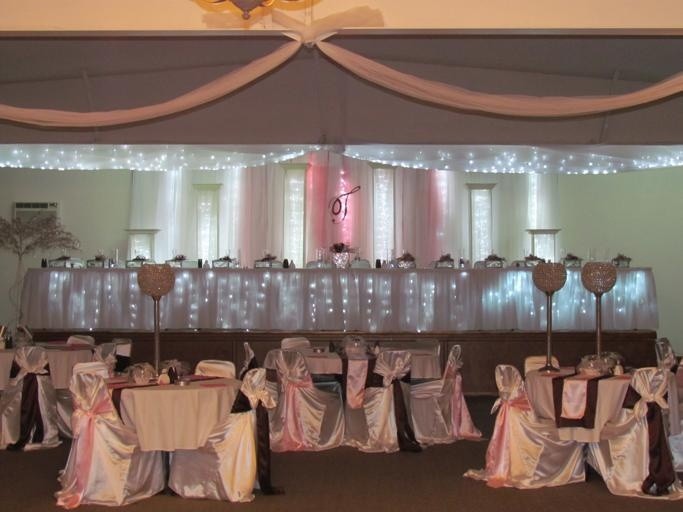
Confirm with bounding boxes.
[613,359,625,375]
[158,369,170,384]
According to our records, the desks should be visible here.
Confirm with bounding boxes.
[525,365,634,446]
[3,345,93,391]
[57,374,242,488]
[262,349,442,381]
[17,268,659,329]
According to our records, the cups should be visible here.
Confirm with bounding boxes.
[6,335,13,349]
[41,246,620,269]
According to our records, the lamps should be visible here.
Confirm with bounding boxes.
[582,261,619,374]
[532,262,566,371]
[138,263,175,379]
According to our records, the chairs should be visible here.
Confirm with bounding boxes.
[92,341,117,375]
[239,341,256,379]
[68,334,92,344]
[55,361,166,508]
[168,368,283,502]
[348,350,413,453]
[273,349,345,452]
[200,359,235,378]
[406,345,478,445]
[112,337,132,372]
[280,336,308,347]
[486,364,585,489]
[2,345,61,449]
[524,355,558,378]
[587,367,669,498]
[57,362,109,440]
